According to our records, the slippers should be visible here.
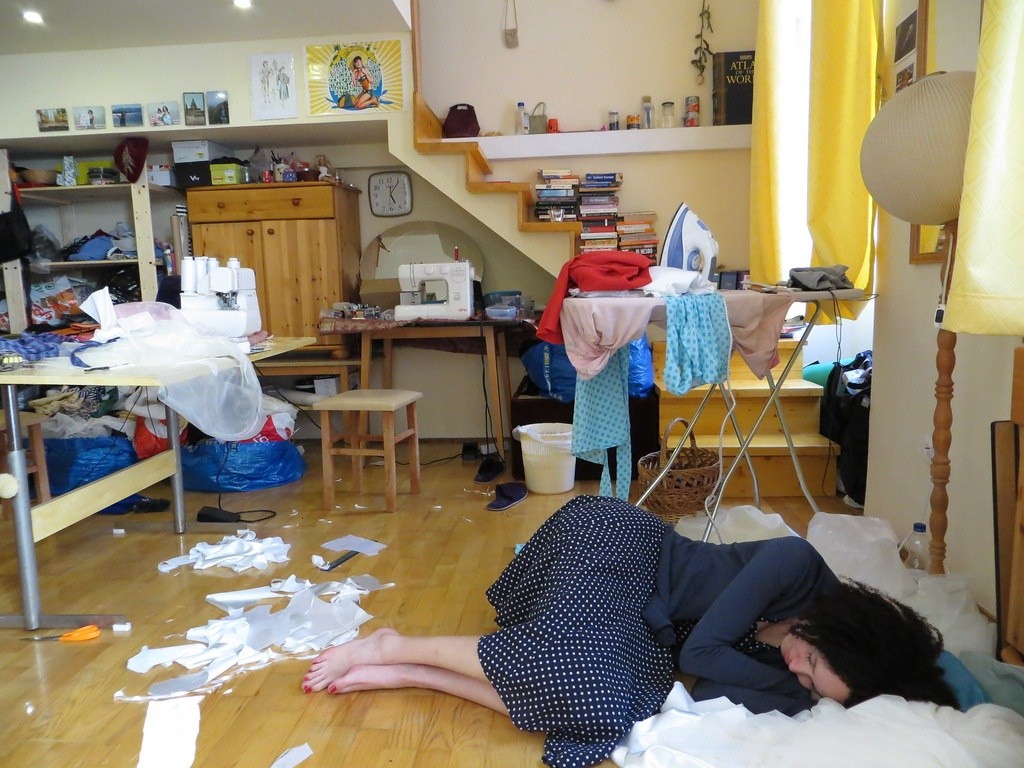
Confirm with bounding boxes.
[474,457,505,483]
[487,481,528,510]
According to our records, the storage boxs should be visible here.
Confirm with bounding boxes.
[175,159,212,188]
[312,369,358,401]
[146,165,179,188]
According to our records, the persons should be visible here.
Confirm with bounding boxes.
[301,495,960,768]
[86,110,96,129]
[157,106,174,125]
[116,112,126,127]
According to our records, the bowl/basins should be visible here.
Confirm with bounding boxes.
[23,170,60,183]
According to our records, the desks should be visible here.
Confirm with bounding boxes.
[318,314,535,462]
[0,336,317,632]
[553,288,862,538]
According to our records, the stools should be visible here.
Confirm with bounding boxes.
[314,387,426,512]
[0,407,50,522]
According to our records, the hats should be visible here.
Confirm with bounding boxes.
[114,136,150,183]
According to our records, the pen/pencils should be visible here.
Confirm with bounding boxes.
[270,150,294,164]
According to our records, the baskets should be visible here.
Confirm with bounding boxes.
[638,418,724,516]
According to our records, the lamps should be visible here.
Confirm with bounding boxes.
[857,68,976,578]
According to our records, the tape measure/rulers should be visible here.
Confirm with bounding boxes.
[321,539,378,571]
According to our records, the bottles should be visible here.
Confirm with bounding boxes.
[241,166,250,183]
[274,164,290,181]
[515,102,529,134]
[898,522,949,574]
[626,114,641,129]
[662,101,674,128]
[283,169,297,182]
[609,111,619,130]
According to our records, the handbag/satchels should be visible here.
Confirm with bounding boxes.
[504,29,519,49]
[529,102,548,134]
[444,104,479,137]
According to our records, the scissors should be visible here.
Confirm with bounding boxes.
[20,625,101,642]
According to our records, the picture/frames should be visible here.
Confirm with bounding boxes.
[909,222,947,265]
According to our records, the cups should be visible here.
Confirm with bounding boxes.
[548,209,565,222]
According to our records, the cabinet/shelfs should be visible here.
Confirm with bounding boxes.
[6,151,187,337]
[185,181,362,360]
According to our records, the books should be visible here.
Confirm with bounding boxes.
[616,210,659,266]
[534,169,579,222]
[578,172,624,254]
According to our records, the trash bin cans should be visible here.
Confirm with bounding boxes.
[519,423,576,495]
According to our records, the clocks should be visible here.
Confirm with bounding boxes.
[368,171,411,218]
